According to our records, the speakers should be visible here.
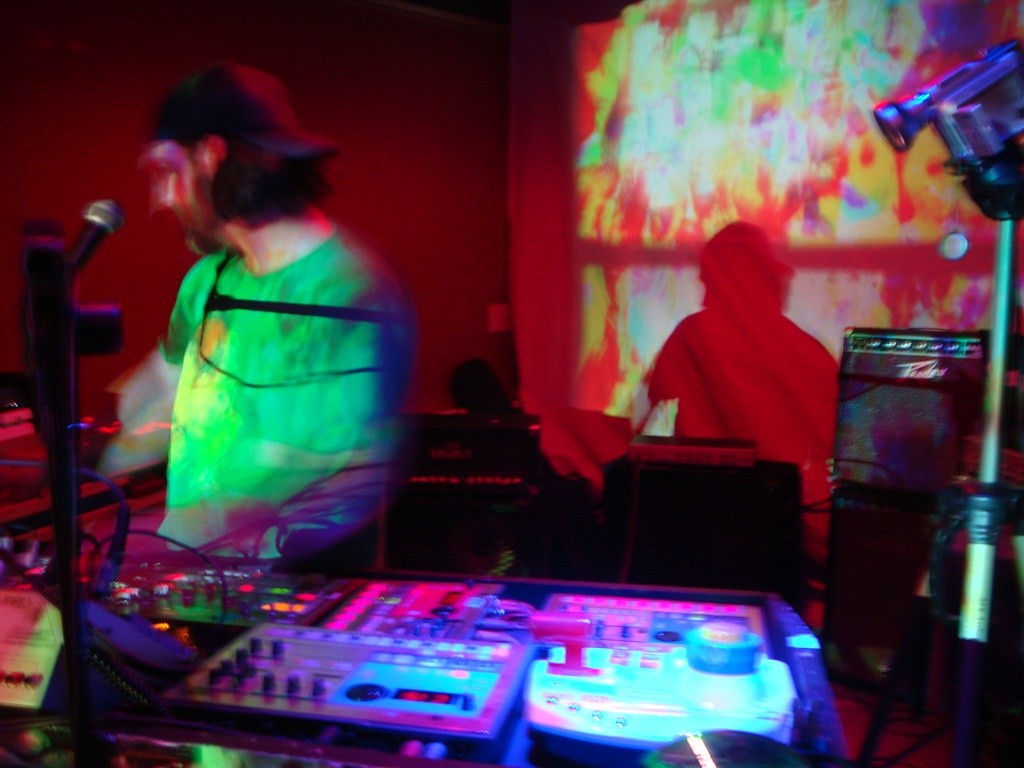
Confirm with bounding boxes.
[376,422,543,586]
[822,485,1024,711]
[833,325,1024,511]
[599,455,808,615]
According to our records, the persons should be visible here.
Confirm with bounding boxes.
[96,66,408,592]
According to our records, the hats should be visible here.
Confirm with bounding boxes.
[157,59,338,157]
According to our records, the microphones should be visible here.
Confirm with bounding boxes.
[67,199,124,271]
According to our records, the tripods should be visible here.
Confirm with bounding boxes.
[860,157,1024,768]
